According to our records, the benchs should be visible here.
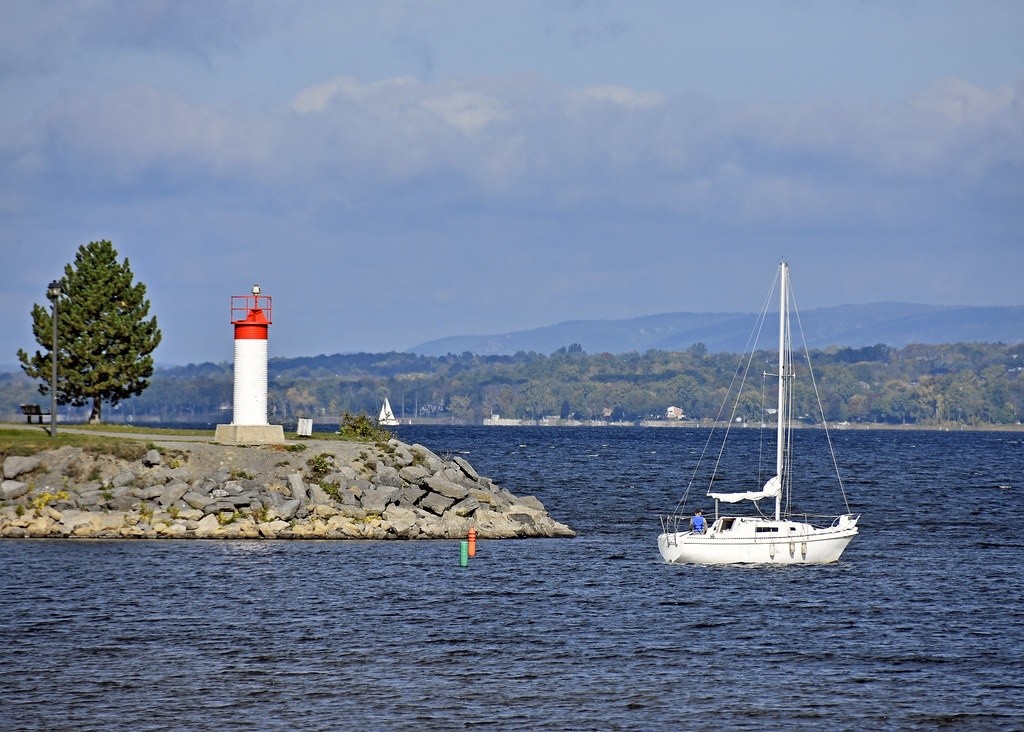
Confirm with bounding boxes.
[18,404,51,424]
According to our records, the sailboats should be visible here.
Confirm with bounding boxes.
[377,396,399,426]
[657,255,862,566]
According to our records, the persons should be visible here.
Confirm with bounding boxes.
[688,509,708,535]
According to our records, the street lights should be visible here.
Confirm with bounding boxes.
[48,280,61,437]
